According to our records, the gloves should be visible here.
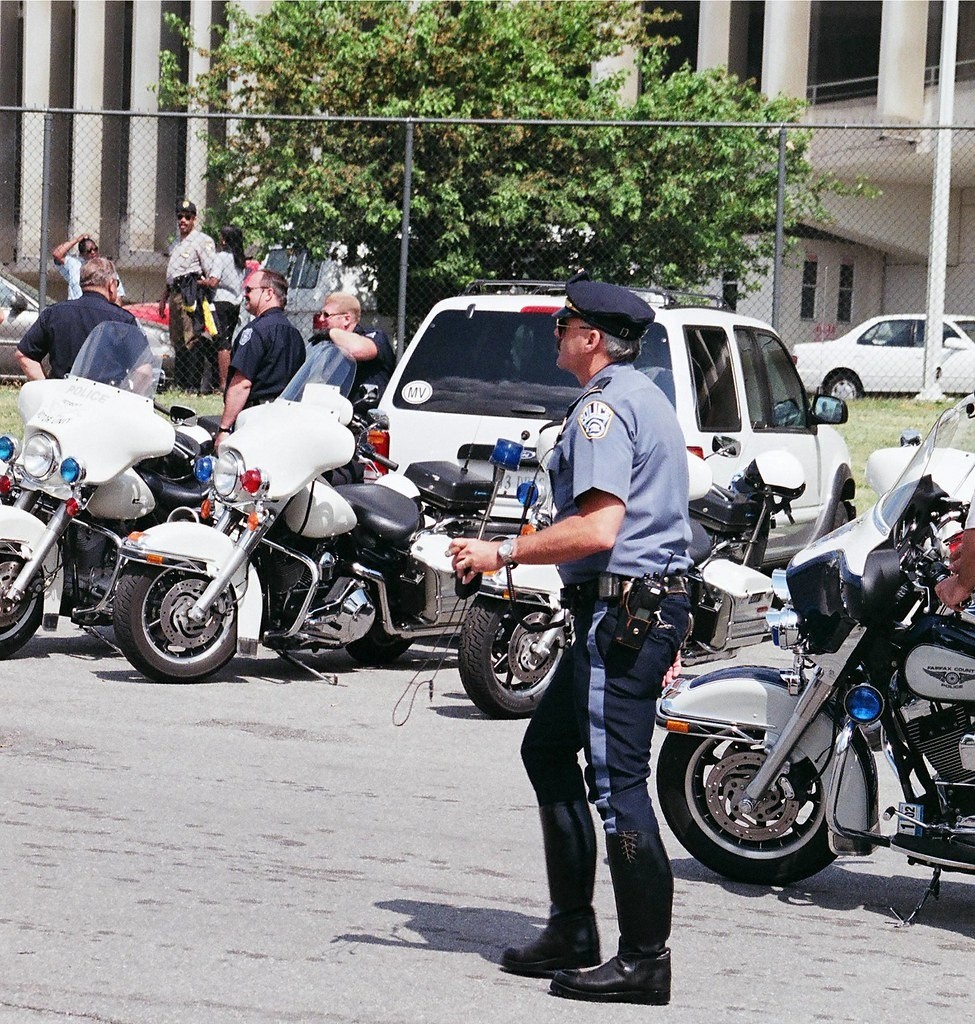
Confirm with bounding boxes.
[307,329,334,346]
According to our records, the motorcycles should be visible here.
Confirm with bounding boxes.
[0,320,813,720]
[651,391,975,930]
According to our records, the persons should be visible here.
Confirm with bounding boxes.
[214,269,307,452]
[935,490,974,613]
[196,226,246,393]
[309,292,396,412]
[53,233,126,308]
[15,258,152,399]
[445,272,692,999]
[159,200,215,390]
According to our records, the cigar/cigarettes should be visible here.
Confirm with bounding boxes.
[446,547,460,557]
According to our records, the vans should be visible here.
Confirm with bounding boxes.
[358,277,858,580]
[235,233,381,360]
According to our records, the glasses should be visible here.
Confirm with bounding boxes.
[87,246,98,253]
[556,318,603,340]
[114,279,120,286]
[177,213,191,220]
[245,286,276,295]
[319,310,348,318]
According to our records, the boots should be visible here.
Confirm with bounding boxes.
[499,800,601,971]
[551,830,674,1006]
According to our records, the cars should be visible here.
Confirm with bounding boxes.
[792,312,975,402]
[0,268,179,393]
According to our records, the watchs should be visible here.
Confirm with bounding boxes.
[498,538,516,567]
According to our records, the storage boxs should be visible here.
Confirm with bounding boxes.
[699,559,775,654]
[424,563,476,625]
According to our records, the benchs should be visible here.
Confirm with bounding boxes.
[429,376,582,408]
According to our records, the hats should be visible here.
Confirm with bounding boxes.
[552,271,655,340]
[175,199,197,215]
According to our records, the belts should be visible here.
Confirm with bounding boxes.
[560,574,688,609]
